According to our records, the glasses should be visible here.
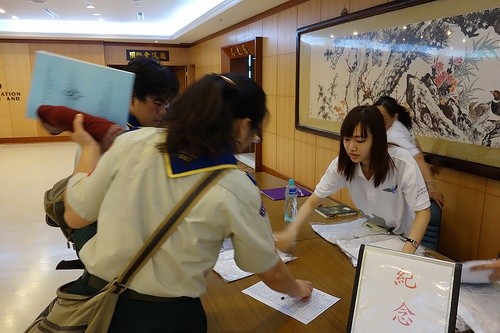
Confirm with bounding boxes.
[250,130,261,144]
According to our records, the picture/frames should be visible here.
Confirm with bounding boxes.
[296,1,500,181]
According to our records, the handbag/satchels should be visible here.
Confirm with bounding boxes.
[44,174,70,227]
[24,278,128,333]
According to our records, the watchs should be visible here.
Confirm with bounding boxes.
[405,239,420,249]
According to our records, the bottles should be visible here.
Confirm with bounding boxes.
[283,179,297,222]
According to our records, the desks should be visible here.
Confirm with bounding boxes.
[202,172,461,333]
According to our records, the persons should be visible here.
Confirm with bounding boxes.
[273,105,431,255]
[73,56,179,260]
[64,72,313,333]
[374,95,445,209]
